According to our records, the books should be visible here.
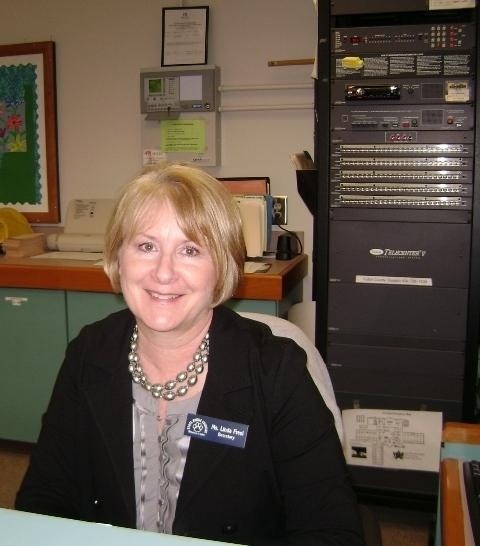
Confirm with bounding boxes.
[290,150,314,170]
[230,193,274,259]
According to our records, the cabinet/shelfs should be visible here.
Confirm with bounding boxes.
[0,287,288,447]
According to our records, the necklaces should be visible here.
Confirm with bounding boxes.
[127,322,212,401]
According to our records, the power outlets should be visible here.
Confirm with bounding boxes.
[272,195,287,225]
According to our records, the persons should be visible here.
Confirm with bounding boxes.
[12,158,368,546]
[270,199,285,225]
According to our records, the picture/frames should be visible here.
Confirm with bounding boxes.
[0,41,60,224]
[161,6,209,67]
